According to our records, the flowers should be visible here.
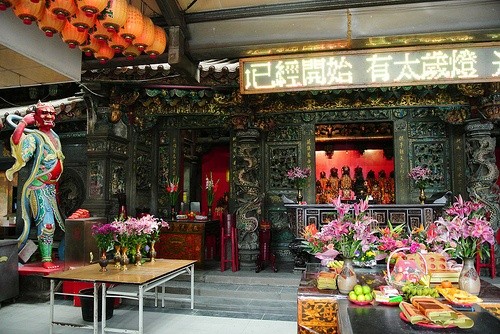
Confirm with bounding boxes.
[286,167,311,188]
[92,214,171,247]
[409,166,431,187]
[159,175,182,205]
[297,194,495,266]
[199,171,220,206]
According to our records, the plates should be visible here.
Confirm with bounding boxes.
[399,312,456,329]
[447,300,473,307]
[347,296,371,306]
[377,302,400,307]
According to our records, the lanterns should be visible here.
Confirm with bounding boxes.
[0,0,167,62]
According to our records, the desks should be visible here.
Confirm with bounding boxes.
[156,218,220,269]
[298,274,500,334]
[42,259,197,334]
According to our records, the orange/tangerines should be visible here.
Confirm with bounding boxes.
[349,284,373,301]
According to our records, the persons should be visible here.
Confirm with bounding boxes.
[315,164,397,205]
[6,100,66,270]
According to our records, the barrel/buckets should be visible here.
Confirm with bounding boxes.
[79,287,114,323]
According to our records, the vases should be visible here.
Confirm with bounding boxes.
[113,244,121,269]
[148,242,157,262]
[171,205,175,220]
[336,257,358,294]
[297,188,303,204]
[134,244,141,266]
[120,248,130,270]
[207,206,212,221]
[99,247,108,272]
[459,257,481,296]
[419,187,426,204]
